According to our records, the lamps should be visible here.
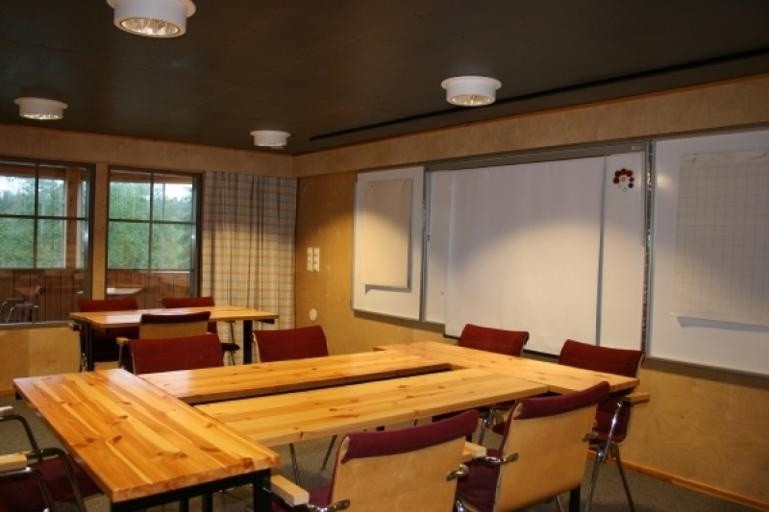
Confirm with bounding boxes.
[247,129,293,149]
[106,0,197,36]
[439,73,503,106]
[13,96,67,120]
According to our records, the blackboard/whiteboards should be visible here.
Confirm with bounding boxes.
[641,120,769,390]
[421,135,654,366]
[352,161,427,327]
